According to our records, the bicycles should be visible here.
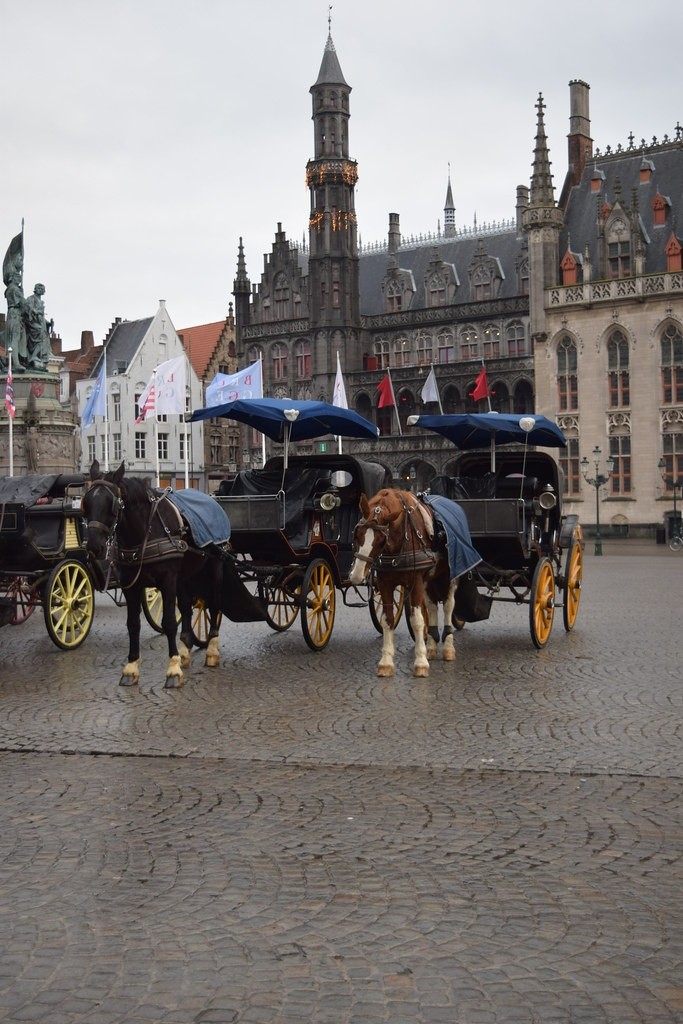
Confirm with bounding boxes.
[668,534,683,551]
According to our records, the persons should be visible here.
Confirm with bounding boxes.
[3,270,28,371]
[20,283,55,374]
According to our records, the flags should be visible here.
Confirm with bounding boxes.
[2,232,23,287]
[80,353,106,437]
[206,360,264,408]
[329,357,349,441]
[421,369,438,404]
[133,355,187,427]
[375,374,397,408]
[3,355,19,419]
[469,367,490,401]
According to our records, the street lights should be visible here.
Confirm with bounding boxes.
[581,445,615,557]
[409,464,418,495]
[657,458,683,538]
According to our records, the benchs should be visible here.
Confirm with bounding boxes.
[0,472,88,519]
[230,468,340,512]
[429,473,539,515]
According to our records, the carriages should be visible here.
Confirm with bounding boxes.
[347,410,584,681]
[77,399,387,691]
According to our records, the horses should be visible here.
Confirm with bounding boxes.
[346,489,472,676]
[81,458,230,689]
